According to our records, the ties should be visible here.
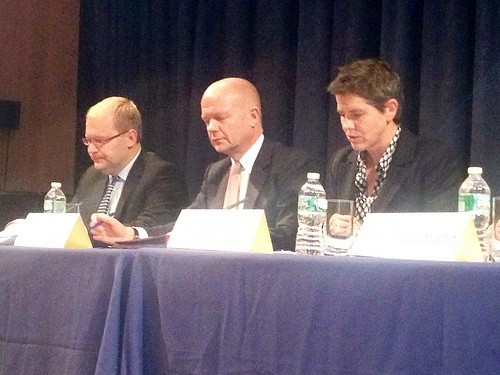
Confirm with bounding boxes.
[97,176,120,214]
[225,162,241,209]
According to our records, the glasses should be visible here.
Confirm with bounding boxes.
[82,130,129,148]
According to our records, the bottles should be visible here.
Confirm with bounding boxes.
[295,173,327,256]
[458,166,490,263]
[43,182,67,213]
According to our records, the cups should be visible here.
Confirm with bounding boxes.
[56,203,79,213]
[492,195,500,262]
[326,199,354,254]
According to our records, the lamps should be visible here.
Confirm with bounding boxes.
[0,101,33,195]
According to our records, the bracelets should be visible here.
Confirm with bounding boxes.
[132,228,139,240]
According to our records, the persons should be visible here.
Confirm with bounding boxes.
[65,97,187,248]
[325,59,464,239]
[90,77,311,251]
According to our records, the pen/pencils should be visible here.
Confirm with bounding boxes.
[87,212,115,231]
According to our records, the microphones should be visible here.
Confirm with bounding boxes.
[225,197,250,209]
